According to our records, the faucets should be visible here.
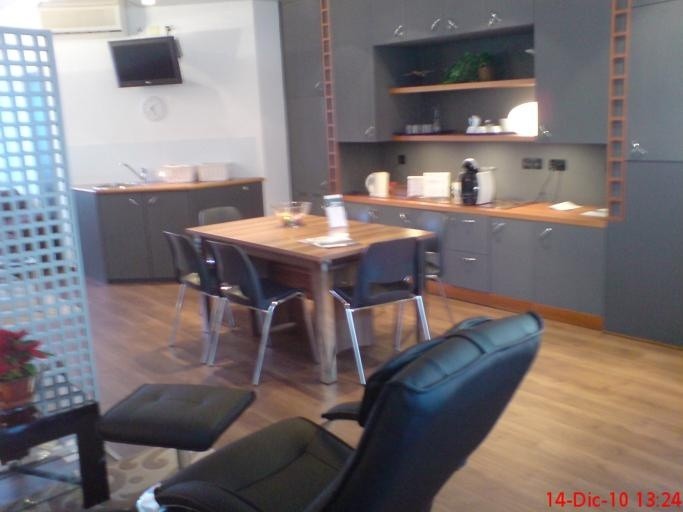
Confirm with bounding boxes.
[118,162,149,183]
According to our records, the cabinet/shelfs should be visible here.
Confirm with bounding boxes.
[96,188,192,282]
[278,0,378,217]
[486,216,605,332]
[481,1,533,31]
[531,0,606,145]
[324,0,419,144]
[372,0,418,46]
[389,77,536,144]
[445,212,486,309]
[343,202,445,300]
[419,1,481,42]
[188,181,264,227]
[605,0,681,160]
[604,160,682,351]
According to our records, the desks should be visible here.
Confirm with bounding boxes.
[0,362,111,510]
[185,212,437,355]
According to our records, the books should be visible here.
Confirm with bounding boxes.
[299,234,358,247]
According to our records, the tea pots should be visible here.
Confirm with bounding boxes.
[364,171,390,198]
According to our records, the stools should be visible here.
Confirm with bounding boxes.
[91,381,258,474]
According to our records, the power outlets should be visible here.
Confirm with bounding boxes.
[548,159,565,171]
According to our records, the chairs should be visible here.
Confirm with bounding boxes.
[198,207,244,226]
[161,229,239,364]
[328,236,431,387]
[134,309,544,512]
[203,236,318,385]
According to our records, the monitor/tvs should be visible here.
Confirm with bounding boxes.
[108,36,183,88]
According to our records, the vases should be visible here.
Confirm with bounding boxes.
[0,374,38,417]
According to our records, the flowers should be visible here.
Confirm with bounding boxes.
[0,329,53,379]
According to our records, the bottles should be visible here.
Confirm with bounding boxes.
[461,158,478,205]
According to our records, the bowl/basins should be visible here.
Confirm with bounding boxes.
[269,201,311,227]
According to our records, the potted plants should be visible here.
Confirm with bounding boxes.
[440,49,502,84]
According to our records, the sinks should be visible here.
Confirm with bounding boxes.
[91,184,143,190]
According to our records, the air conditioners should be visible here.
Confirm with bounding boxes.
[38,1,128,39]
